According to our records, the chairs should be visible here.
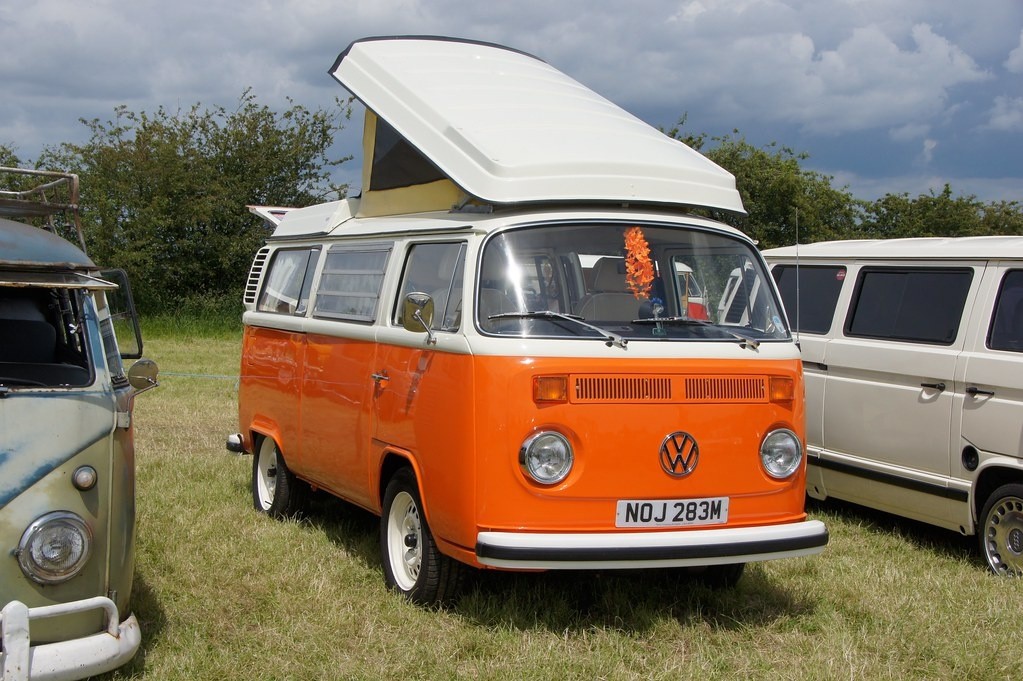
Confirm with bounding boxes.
[429,245,522,332]
[577,257,654,321]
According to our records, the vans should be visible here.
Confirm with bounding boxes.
[719,236,1022,581]
[505,250,709,324]
[1,167,159,681]
[716,267,744,320]
[226,35,830,618]
